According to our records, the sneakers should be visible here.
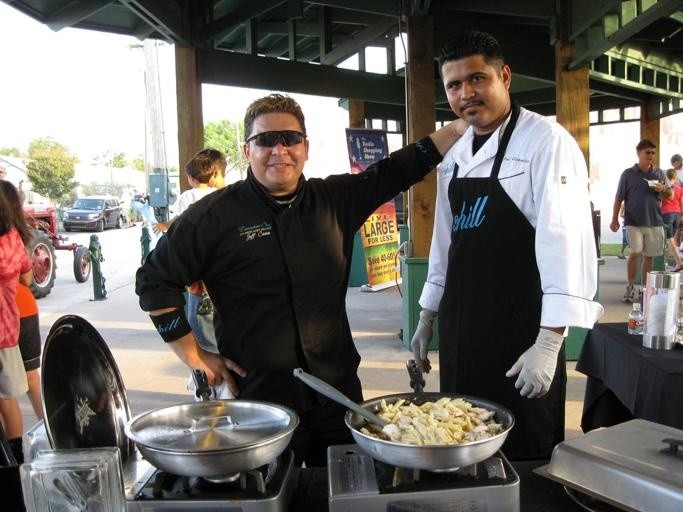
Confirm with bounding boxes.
[623,285,635,302]
[197,295,213,314]
[670,264,683,271]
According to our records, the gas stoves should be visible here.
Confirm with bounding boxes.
[124,449,296,512]
[325,444,522,512]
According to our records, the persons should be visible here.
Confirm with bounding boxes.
[609,139,676,303]
[122,187,154,227]
[12,268,46,423]
[187,146,232,401]
[667,154,683,187]
[132,91,469,468]
[0,166,8,179]
[151,154,219,235]
[659,169,682,272]
[409,29,606,460]
[0,181,27,463]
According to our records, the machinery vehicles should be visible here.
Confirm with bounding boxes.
[16,177,91,299]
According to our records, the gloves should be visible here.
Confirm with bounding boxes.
[505,328,564,400]
[411,310,434,373]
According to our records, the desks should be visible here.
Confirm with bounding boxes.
[571,319,682,442]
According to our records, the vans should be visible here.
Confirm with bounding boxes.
[61,196,124,231]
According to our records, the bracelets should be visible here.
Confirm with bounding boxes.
[149,308,194,343]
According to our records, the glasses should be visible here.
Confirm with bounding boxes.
[247,131,307,147]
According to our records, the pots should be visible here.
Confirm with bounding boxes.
[343,359,517,472]
[121,367,300,479]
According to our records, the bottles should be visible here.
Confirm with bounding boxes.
[627,302,645,336]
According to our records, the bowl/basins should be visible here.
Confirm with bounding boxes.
[647,179,658,187]
